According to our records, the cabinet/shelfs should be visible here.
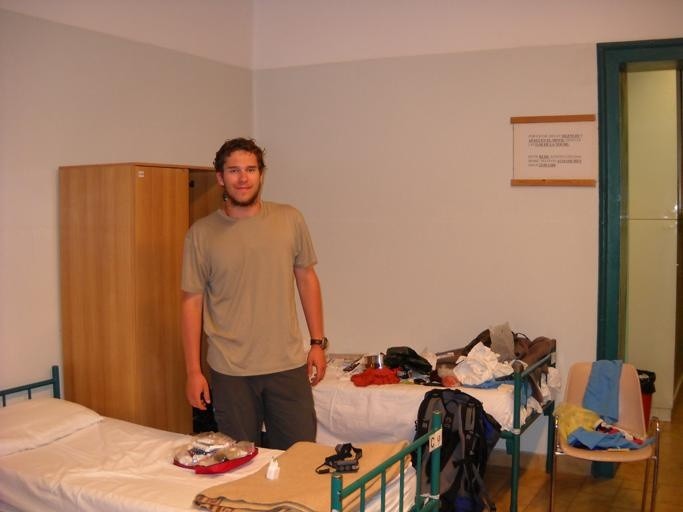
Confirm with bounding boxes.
[55,163,228,434]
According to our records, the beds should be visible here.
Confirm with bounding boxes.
[3,366,441,511]
[195,348,557,509]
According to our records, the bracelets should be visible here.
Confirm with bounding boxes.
[309,336,328,349]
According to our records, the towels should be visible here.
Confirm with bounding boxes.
[582,358,624,425]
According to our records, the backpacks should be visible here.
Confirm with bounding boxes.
[410,387,502,512]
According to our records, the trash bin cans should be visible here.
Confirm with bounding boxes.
[637,370,656,432]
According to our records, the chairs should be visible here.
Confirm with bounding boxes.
[547,361,666,510]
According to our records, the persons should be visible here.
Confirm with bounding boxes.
[180,138,326,452]
